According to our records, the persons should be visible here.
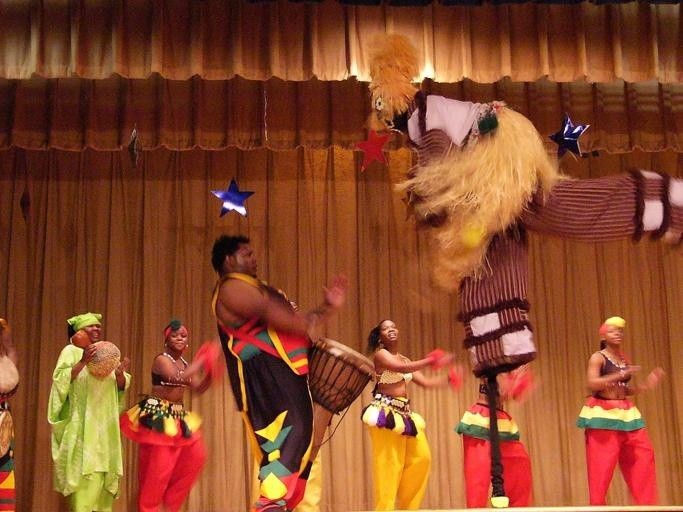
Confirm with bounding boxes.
[456,365,535,508]
[361,319,461,511]
[47,312,132,512]
[211,234,349,512]
[0,318,20,512]
[137,320,219,512]
[575,316,666,505]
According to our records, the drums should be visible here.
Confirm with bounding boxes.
[298,337,375,480]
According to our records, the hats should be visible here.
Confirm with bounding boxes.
[66,312,103,331]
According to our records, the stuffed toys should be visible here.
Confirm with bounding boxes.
[369,34,682,373]
